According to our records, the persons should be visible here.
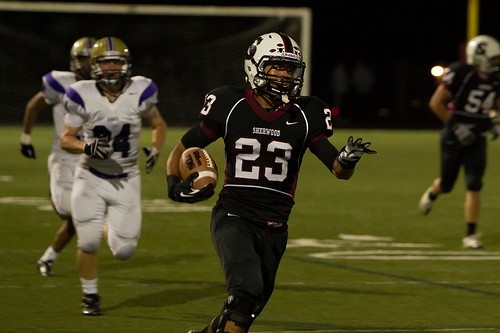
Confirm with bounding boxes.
[419,35,500,249]
[61,37,167,315]
[20,37,98,276]
[165,33,378,333]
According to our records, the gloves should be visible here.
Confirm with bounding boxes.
[20,141,37,159]
[83,139,112,160]
[452,123,477,144]
[171,172,214,204]
[142,144,158,174]
[487,124,500,141]
[336,136,376,179]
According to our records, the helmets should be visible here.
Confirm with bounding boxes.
[89,37,133,79]
[244,33,304,94]
[466,35,500,73]
[70,39,92,69]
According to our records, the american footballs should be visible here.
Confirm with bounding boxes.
[178,147,218,192]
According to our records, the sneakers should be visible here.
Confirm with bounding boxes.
[419,186,436,215]
[37,258,56,276]
[80,292,103,315]
[462,232,484,248]
